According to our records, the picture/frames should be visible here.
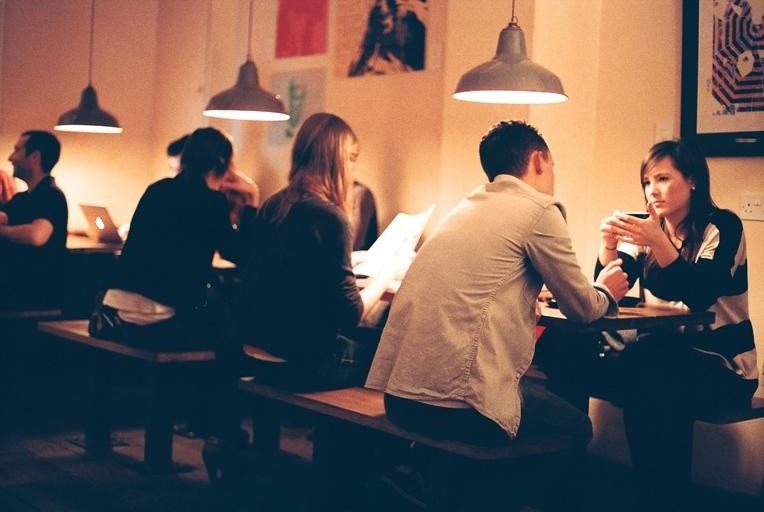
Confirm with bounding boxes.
[679,0,764,158]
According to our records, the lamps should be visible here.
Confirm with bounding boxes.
[200,2,292,119]
[52,1,122,136]
[448,0,567,103]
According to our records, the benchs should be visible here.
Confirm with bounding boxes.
[38,317,214,474]
[237,375,536,512]
[589,396,762,512]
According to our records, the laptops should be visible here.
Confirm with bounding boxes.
[78,203,126,244]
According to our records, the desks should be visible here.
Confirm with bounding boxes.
[46,228,123,257]
[213,258,715,406]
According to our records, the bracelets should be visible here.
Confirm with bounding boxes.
[606,246,616,250]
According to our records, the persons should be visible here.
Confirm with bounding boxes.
[594,140,759,474]
[0,130,68,311]
[363,120,630,447]
[347,0,426,77]
